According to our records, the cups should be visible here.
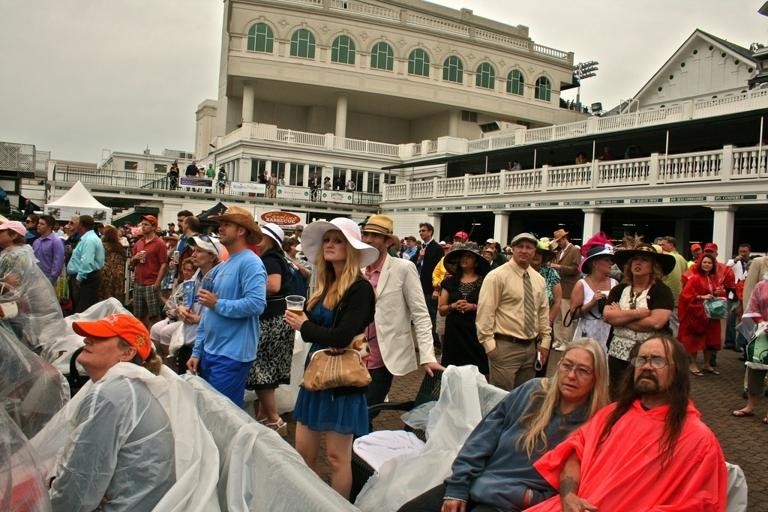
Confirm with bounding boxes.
[284,293,308,316]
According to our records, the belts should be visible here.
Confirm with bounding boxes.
[494,333,539,345]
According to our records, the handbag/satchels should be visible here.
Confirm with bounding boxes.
[304,348,373,392]
[55,276,70,303]
[703,297,729,319]
[598,295,607,314]
[745,323,768,369]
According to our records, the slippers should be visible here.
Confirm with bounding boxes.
[732,410,754,416]
[763,416,768,424]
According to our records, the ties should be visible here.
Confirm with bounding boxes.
[524,271,535,336]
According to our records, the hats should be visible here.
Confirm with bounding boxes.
[301,217,380,268]
[443,243,490,276]
[552,228,569,242]
[261,223,284,251]
[455,231,468,241]
[614,244,676,275]
[690,244,701,252]
[581,242,615,274]
[0,220,26,237]
[536,241,557,263]
[208,205,263,245]
[511,232,538,247]
[405,236,416,242]
[72,314,152,360]
[140,215,157,226]
[189,232,221,258]
[704,243,718,252]
[362,215,393,238]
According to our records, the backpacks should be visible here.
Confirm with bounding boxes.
[268,249,308,300]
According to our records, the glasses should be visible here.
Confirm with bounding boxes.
[26,220,34,224]
[193,232,219,254]
[630,356,675,369]
[419,229,429,232]
[557,360,593,376]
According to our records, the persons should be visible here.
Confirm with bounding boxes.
[509,159,522,171]
[166,158,356,203]
[1,184,768,511]
[574,145,637,163]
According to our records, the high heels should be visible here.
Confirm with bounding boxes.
[256,417,288,438]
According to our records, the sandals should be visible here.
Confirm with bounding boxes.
[712,368,720,375]
[689,367,704,377]
